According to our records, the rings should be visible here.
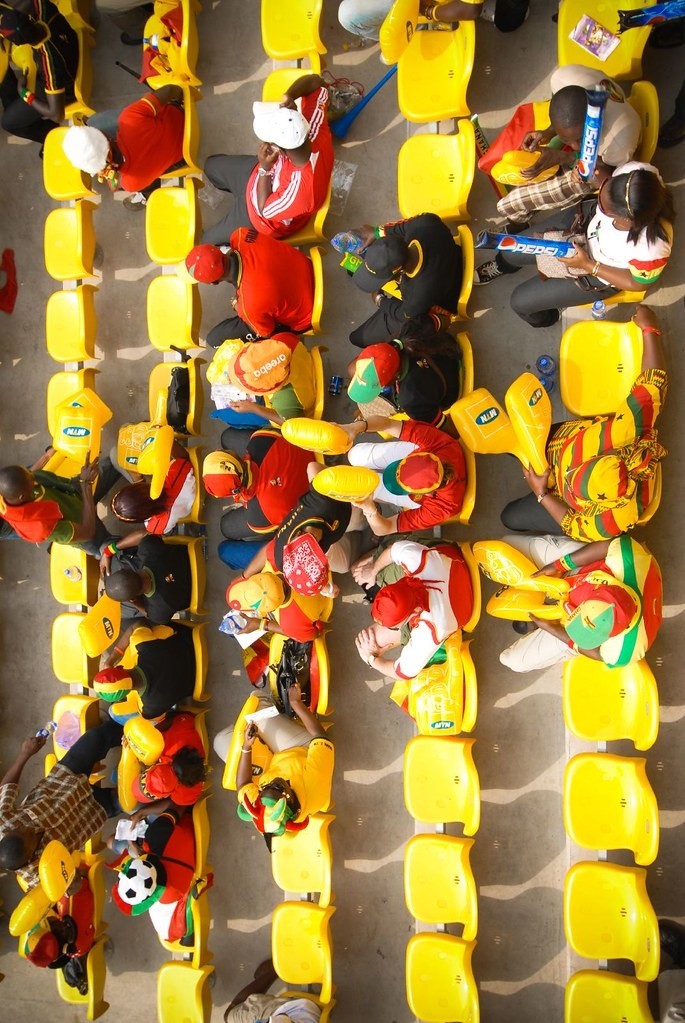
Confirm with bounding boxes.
[523,477,526,479]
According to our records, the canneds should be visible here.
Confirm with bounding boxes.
[329,375,343,396]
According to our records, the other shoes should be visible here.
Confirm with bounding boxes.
[252,960,280,978]
[511,620,538,634]
[123,193,145,211]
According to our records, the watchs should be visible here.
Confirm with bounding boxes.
[366,653,378,671]
[257,167,273,177]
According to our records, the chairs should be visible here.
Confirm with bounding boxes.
[44,200,99,280]
[64,27,96,119]
[558,0,660,1023]
[156,891,216,1023]
[175,707,212,876]
[261,0,327,102]
[268,569,336,1023]
[144,0,203,264]
[57,775,108,1021]
[43,113,97,201]
[147,275,206,525]
[52,612,102,689]
[397,16,481,1023]
[162,535,211,701]
[276,176,333,469]
[53,694,101,761]
[47,368,102,495]
[50,541,100,607]
[46,283,101,362]
[49,0,95,31]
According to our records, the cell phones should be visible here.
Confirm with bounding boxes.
[267,143,284,163]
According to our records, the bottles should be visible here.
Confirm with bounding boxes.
[590,299,608,322]
[538,377,556,394]
[331,231,369,255]
[194,525,208,563]
[143,35,159,50]
[36,720,58,745]
[218,612,260,634]
[536,355,556,376]
[64,566,82,582]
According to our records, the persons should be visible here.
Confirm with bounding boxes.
[649,17,685,149]
[338,0,485,65]
[183,65,669,837]
[0,0,185,211]
[0,420,207,969]
[224,958,322,1023]
[658,920,685,1023]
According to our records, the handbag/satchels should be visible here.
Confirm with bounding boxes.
[166,366,191,428]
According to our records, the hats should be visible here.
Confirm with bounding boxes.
[346,342,400,402]
[383,451,444,496]
[238,794,311,854]
[174,243,226,283]
[352,237,404,292]
[112,853,166,915]
[61,127,109,175]
[228,339,292,393]
[561,569,636,650]
[18,917,58,967]
[93,668,132,702]
[227,571,285,613]
[132,763,177,804]
[254,106,309,148]
[371,578,415,627]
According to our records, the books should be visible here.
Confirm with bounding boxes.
[569,14,621,62]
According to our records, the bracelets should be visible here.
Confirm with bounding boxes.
[537,489,555,503]
[375,294,385,308]
[642,327,662,336]
[103,542,119,557]
[260,619,270,631]
[553,554,578,573]
[19,88,36,106]
[241,570,245,580]
[592,261,601,277]
[363,508,378,516]
[114,646,124,654]
[354,416,369,434]
[131,479,143,484]
[79,480,94,484]
[425,4,441,22]
[242,750,252,753]
[567,639,581,655]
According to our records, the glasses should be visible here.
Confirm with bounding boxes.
[597,177,618,217]
[260,784,290,800]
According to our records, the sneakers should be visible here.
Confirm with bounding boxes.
[473,259,508,284]
[476,226,510,239]
